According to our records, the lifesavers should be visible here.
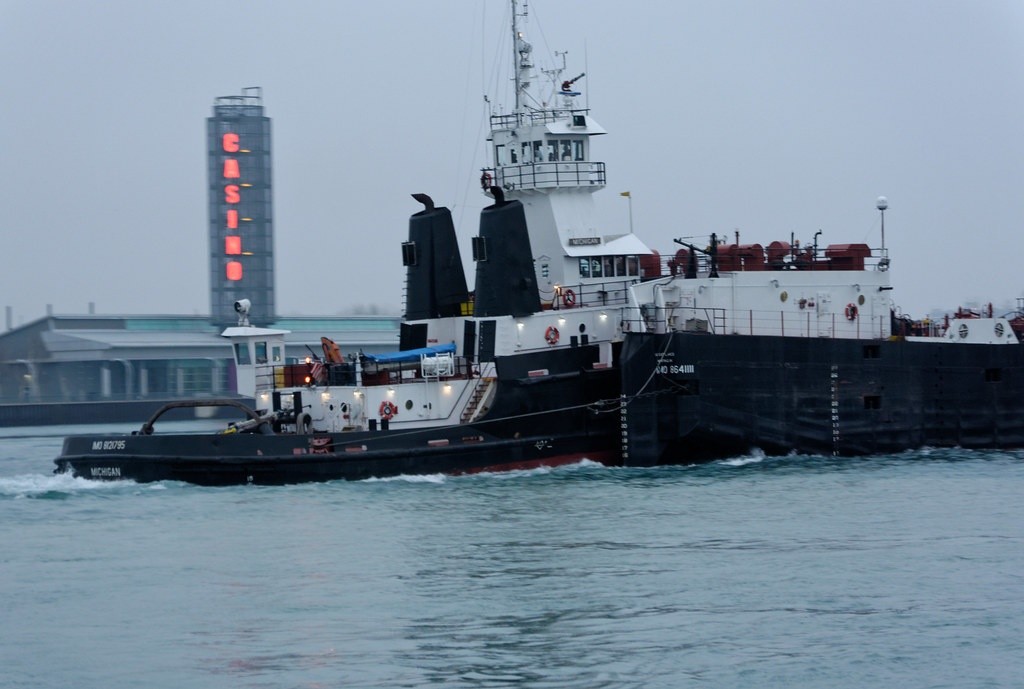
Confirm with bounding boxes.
[563,289,576,308]
[845,303,858,321]
[379,401,395,421]
[544,326,559,346]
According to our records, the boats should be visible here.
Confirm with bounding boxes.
[52,1,1024,487]
